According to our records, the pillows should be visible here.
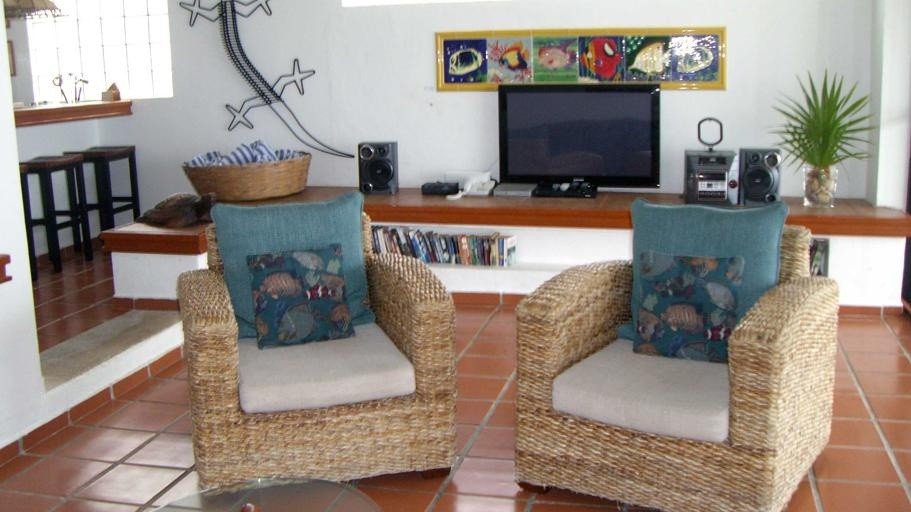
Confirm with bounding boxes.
[631,198,791,364]
[247,244,356,349]
[210,189,378,340]
[632,251,744,363]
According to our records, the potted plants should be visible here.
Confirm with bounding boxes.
[771,67,879,209]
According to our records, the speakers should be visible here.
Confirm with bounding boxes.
[739,148,781,206]
[358,141,399,194]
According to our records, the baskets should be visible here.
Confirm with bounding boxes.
[182,148,313,203]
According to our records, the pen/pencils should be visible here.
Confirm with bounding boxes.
[52,74,87,103]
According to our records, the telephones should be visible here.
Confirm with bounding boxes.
[462,173,495,195]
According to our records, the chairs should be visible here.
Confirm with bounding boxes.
[514,223,839,512]
[175,209,458,486]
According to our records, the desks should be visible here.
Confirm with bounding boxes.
[98,186,911,309]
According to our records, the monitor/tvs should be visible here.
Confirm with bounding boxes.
[498,84,660,198]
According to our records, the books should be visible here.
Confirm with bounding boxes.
[371,224,520,267]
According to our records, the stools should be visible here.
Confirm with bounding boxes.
[63,145,140,261]
[19,155,92,282]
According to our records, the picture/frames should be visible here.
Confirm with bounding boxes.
[7,40,16,75]
[436,27,727,91]
